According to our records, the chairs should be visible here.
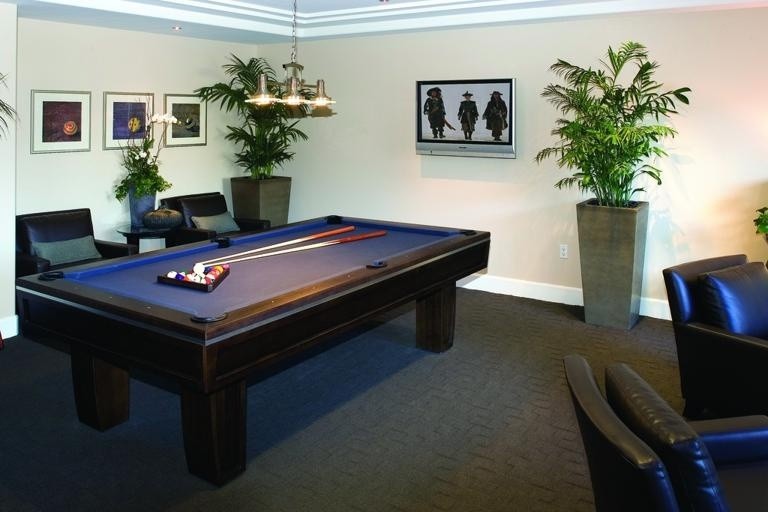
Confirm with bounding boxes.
[663,254,768,418]
[161,192,271,247]
[564,354,768,512]
[16,208,137,315]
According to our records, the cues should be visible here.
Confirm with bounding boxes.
[207,229,388,266]
[193,225,356,266]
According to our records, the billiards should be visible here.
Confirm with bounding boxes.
[165,263,229,285]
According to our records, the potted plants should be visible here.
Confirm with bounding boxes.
[533,40,692,330]
[193,52,309,226]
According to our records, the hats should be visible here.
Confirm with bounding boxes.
[490,91,504,96]
[426,87,442,96]
[462,91,473,96]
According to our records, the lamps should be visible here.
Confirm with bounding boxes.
[244,0,337,107]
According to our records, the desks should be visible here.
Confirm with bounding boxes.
[15,215,490,484]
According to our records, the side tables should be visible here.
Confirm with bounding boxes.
[117,224,172,244]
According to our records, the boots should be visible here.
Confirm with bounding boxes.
[432,128,445,138]
[463,130,473,140]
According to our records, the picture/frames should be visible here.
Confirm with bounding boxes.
[102,91,154,151]
[164,94,207,147]
[31,89,91,154]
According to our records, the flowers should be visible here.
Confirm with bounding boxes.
[112,95,181,203]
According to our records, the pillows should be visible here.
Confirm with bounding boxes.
[18,211,93,255]
[176,194,227,228]
[699,260,768,335]
[191,211,240,234]
[31,236,102,266]
[605,361,728,511]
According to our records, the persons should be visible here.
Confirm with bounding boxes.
[457,90,479,140]
[423,87,455,139]
[482,91,508,141]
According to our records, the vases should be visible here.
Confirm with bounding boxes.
[130,184,156,226]
